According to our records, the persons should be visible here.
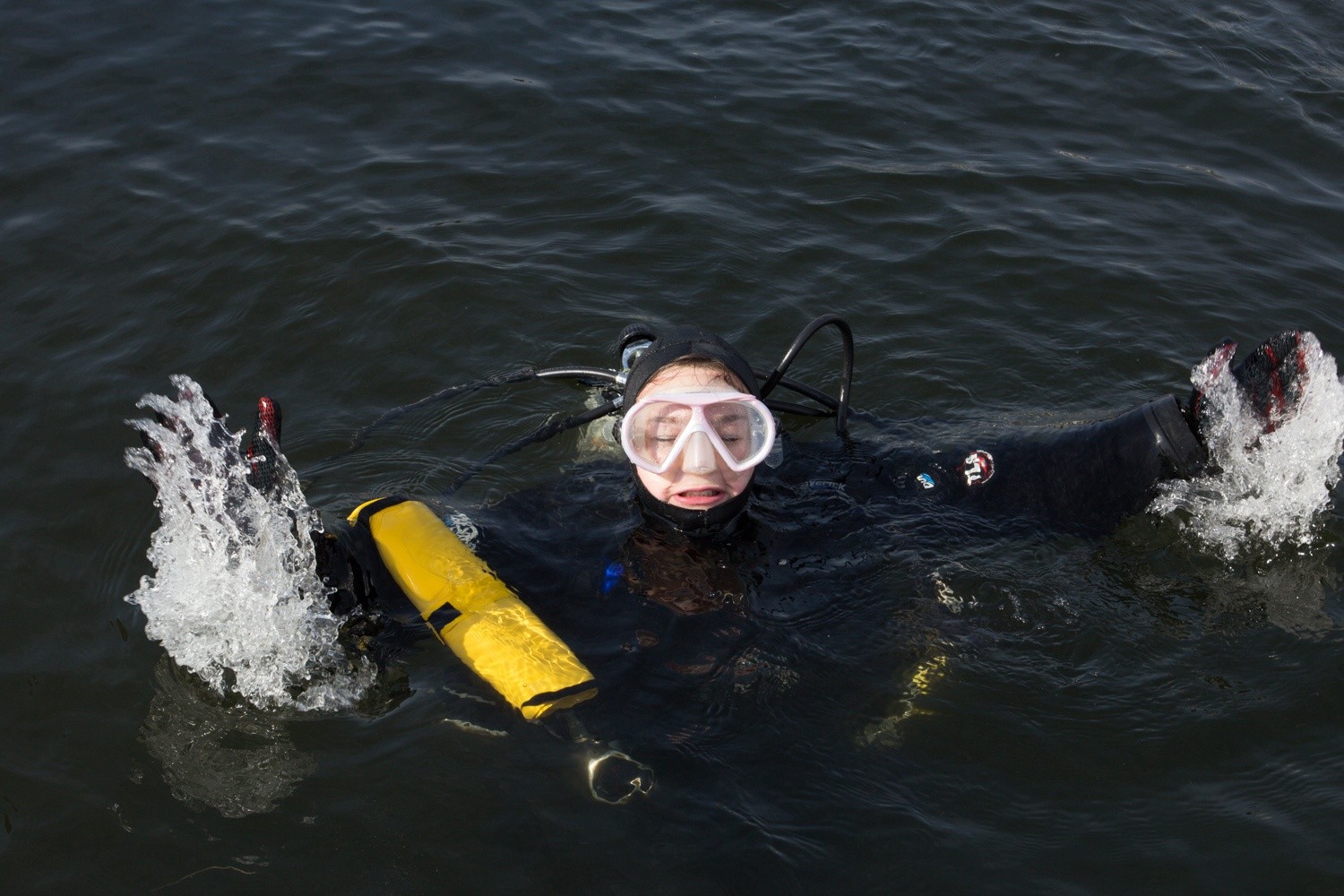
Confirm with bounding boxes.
[138,322,1303,686]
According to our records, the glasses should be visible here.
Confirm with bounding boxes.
[617,389,776,473]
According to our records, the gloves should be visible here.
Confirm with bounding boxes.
[1180,328,1306,441]
[144,388,281,498]
